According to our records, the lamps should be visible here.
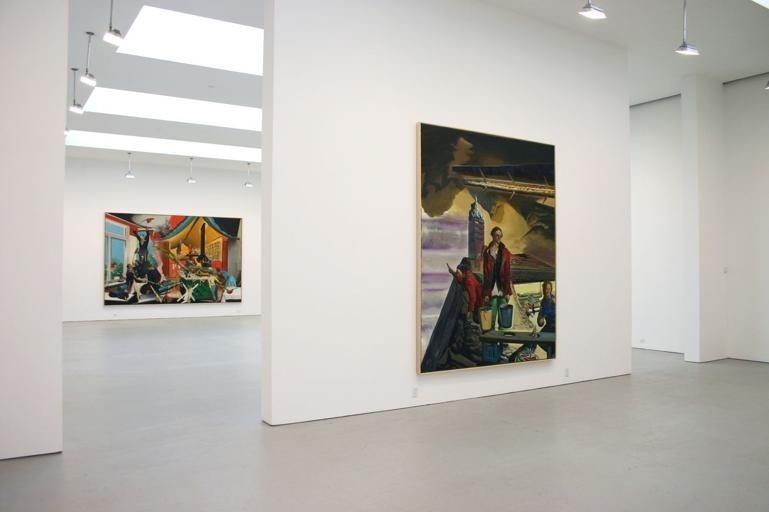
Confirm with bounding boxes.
[123,153,137,180]
[185,158,197,184]
[79,32,98,90]
[765,80,769,90]
[673,1,701,56]
[103,0,125,50]
[242,165,254,190]
[577,0,608,21]
[69,66,84,117]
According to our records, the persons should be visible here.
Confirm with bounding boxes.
[537,279,555,358]
[478,245,488,272]
[481,226,514,331]
[446,256,483,322]
